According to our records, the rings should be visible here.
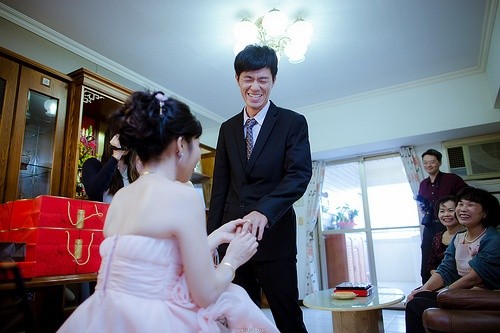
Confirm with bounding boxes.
[246,219,251,222]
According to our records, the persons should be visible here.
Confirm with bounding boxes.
[82,111,140,204]
[55,90,282,333]
[404,187,500,333]
[207,44,312,333]
[415,149,470,285]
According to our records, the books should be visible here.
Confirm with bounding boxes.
[332,282,374,296]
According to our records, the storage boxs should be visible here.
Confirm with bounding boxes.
[0,194,110,281]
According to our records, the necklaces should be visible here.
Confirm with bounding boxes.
[464,227,486,243]
[117,165,126,170]
[141,171,161,174]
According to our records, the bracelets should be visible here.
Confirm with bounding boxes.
[222,262,236,281]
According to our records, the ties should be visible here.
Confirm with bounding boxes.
[243,119,257,160]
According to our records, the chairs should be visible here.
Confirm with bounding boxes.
[420,286,500,333]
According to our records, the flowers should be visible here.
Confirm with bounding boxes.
[77,125,102,169]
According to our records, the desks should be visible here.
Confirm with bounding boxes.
[0,272,99,333]
[302,287,406,333]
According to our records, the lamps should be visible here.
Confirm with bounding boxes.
[231,7,313,65]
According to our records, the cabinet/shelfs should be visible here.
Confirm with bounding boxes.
[325,233,366,288]
[0,46,72,203]
[57,66,134,200]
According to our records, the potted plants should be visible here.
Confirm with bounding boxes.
[330,202,359,229]
